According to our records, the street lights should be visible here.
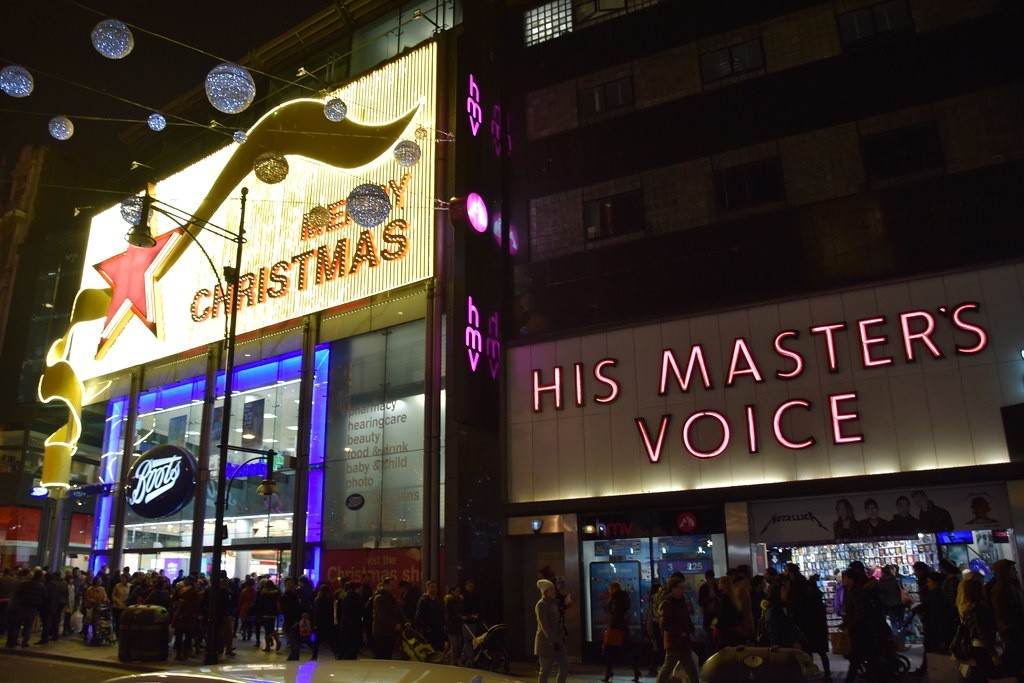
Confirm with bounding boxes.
[31,486,67,572]
[125,187,280,666]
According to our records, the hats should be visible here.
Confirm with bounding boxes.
[343,581,362,594]
[536,579,554,593]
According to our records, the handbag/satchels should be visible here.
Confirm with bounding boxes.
[299,617,312,638]
[604,630,625,648]
[70,608,84,634]
[831,625,853,656]
[32,614,40,631]
[896,582,912,606]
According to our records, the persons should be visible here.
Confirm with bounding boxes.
[833,491,954,539]
[644,559,1024,683]
[2,562,484,669]
[536,565,567,675]
[533,579,569,683]
[600,580,642,683]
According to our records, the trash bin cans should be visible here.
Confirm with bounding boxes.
[116,603,172,664]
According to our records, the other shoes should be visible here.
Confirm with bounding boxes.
[168,629,305,663]
[905,665,926,680]
[0,628,93,648]
[822,676,834,683]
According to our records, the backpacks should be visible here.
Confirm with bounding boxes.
[735,611,755,641]
[755,604,778,647]
[949,620,978,660]
[673,649,701,680]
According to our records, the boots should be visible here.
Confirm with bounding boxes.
[648,650,660,675]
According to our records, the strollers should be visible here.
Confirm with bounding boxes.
[855,605,919,674]
[461,613,510,674]
[87,601,116,645]
[401,626,450,665]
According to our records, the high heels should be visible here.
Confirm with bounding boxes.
[601,672,614,682]
[631,671,642,682]
